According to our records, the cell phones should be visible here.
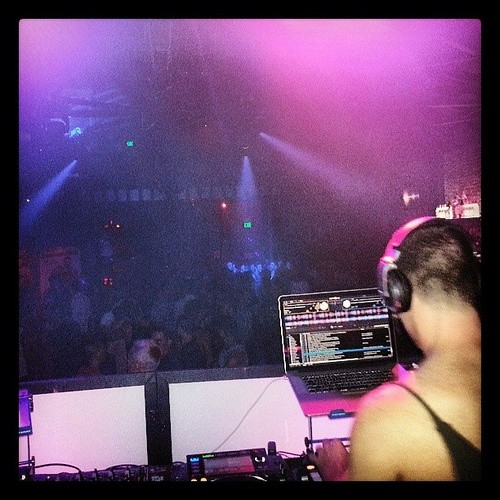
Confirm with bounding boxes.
[19,389,33,436]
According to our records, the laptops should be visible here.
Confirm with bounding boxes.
[277,285,411,416]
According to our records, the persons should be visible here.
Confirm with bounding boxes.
[77,264,282,379]
[308,215,482,482]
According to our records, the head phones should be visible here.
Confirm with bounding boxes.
[377,216,483,315]
